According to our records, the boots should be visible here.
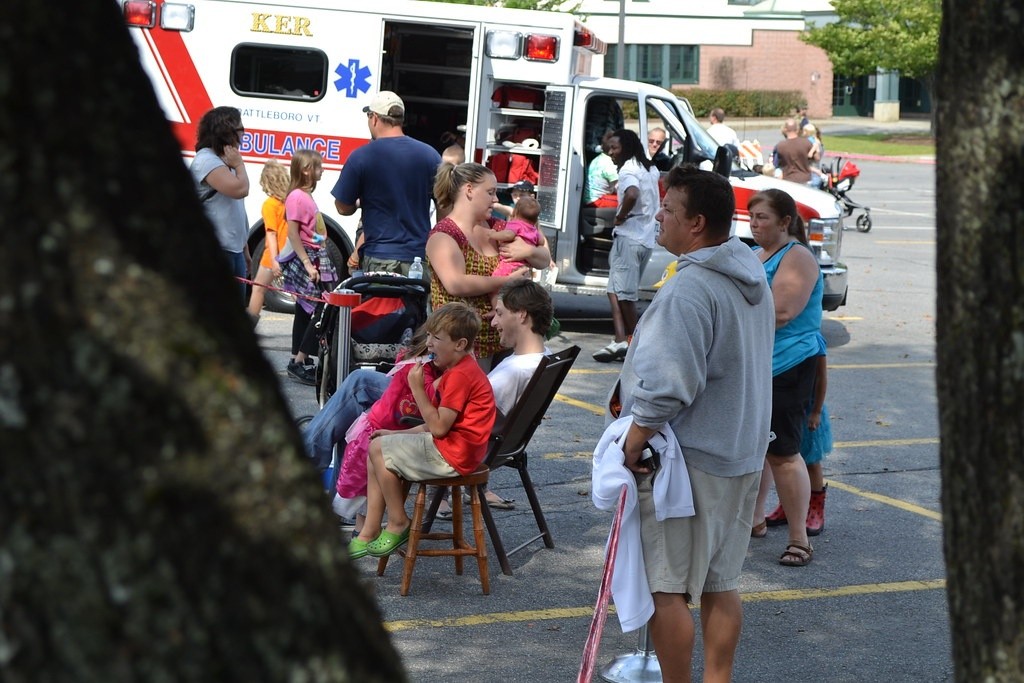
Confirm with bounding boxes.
[807,483,827,535]
[765,500,787,526]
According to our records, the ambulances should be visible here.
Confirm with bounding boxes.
[117,0,852,335]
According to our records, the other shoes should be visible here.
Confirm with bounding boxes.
[463,493,516,509]
[428,499,453,518]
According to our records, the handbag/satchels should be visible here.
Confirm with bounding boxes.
[490,81,543,110]
[486,153,539,185]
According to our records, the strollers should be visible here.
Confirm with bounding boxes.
[808,155,873,233]
[294,270,436,432]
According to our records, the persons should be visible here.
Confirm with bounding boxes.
[188,106,253,308]
[345,302,496,560]
[645,127,666,158]
[764,331,834,537]
[330,91,444,276]
[592,130,661,362]
[482,197,546,318]
[604,167,776,683]
[773,105,823,186]
[706,108,743,164]
[426,164,552,520]
[245,160,292,330]
[303,280,557,531]
[583,128,620,209]
[275,149,336,386]
[348,139,537,272]
[746,189,825,566]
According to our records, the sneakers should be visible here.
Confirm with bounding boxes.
[287,355,316,386]
[592,340,628,363]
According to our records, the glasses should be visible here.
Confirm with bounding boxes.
[367,110,379,119]
[234,126,245,132]
[648,138,663,146]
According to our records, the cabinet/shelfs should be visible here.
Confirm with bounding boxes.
[480,76,567,192]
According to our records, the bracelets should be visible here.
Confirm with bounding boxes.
[615,214,622,219]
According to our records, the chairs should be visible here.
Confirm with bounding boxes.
[420,345,582,576]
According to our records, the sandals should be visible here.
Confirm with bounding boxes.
[779,541,813,566]
[750,520,768,537]
[366,518,412,557]
[346,537,378,558]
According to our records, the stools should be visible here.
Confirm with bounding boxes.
[377,464,489,596]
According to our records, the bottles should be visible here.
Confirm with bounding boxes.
[408,257,423,279]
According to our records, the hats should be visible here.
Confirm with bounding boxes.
[363,91,405,122]
[508,181,534,194]
